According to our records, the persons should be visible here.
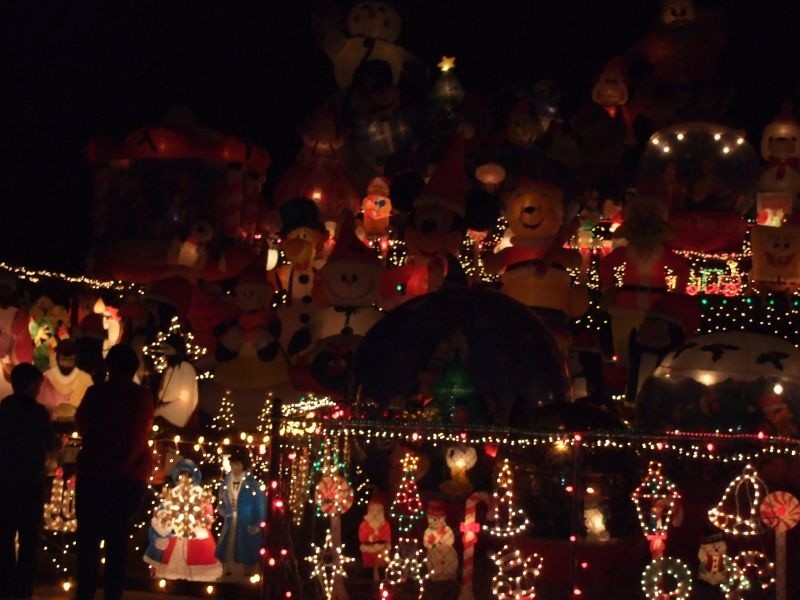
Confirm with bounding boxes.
[143,457,224,583]
[37,339,96,425]
[71,345,154,592]
[213,450,267,589]
[150,331,200,434]
[0,362,63,600]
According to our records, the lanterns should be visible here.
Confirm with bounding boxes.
[0,0,800,600]
